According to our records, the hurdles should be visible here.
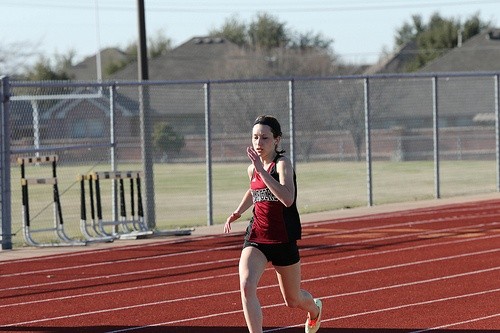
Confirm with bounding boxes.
[18,155,195,247]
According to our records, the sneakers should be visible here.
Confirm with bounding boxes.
[305,298,322,333]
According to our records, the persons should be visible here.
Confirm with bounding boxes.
[223,116,322,333]
